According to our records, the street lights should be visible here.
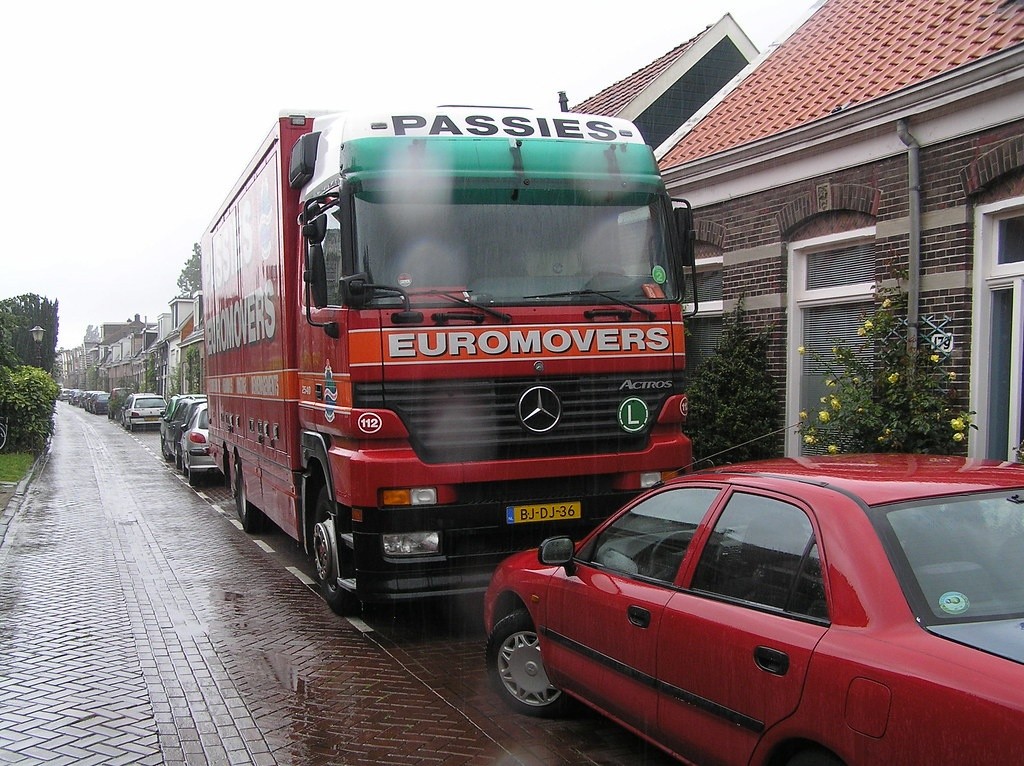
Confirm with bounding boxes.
[30,323,47,368]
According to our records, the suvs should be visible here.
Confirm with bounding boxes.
[162,394,223,488]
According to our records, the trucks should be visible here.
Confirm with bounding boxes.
[204,92,699,614]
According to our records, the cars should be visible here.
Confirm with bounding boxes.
[55,384,167,431]
[479,457,1024,765]
